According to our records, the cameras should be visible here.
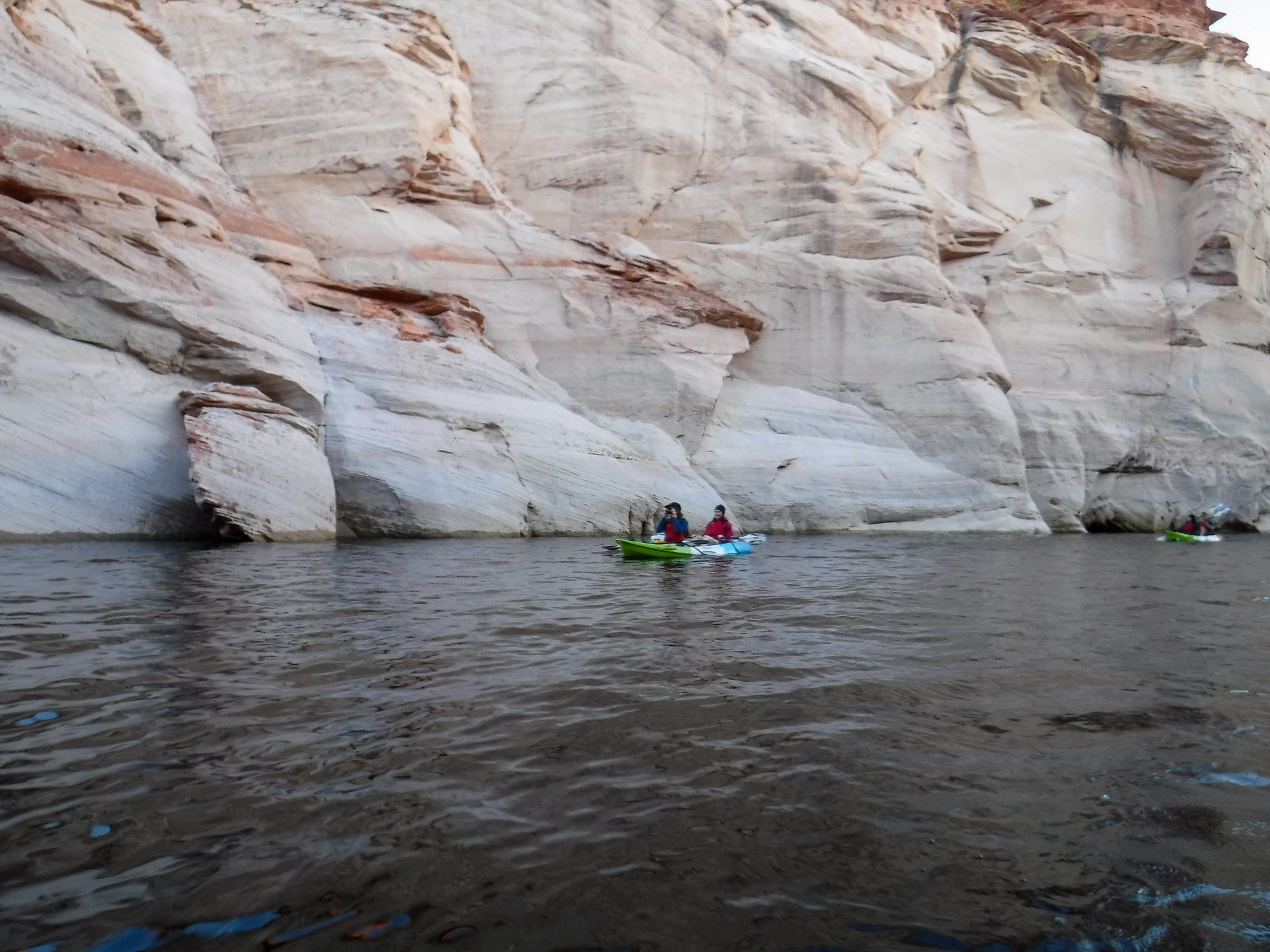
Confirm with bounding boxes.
[666,508,672,514]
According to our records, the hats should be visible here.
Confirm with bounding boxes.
[664,502,681,511]
[715,504,725,515]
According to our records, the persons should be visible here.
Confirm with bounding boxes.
[656,502,687,544]
[682,504,732,547]
[1182,512,1214,535]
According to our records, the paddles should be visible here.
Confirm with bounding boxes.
[600,540,709,550]
[1156,503,1230,541]
[650,532,767,545]
